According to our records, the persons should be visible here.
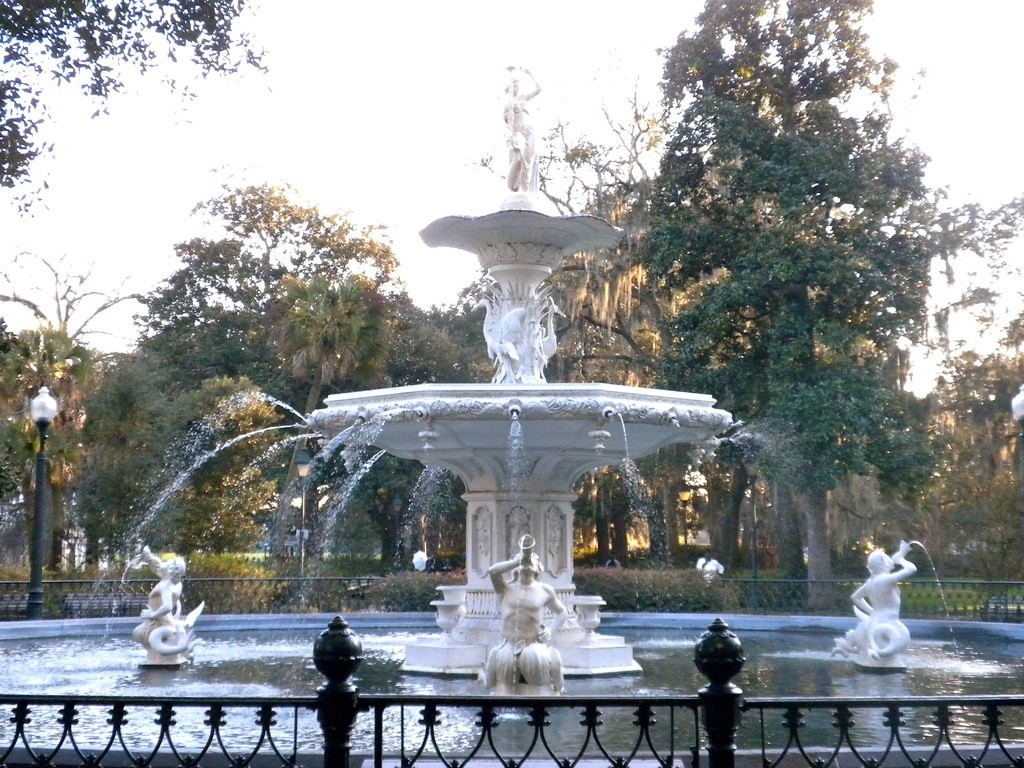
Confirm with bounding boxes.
[133,556,205,660]
[830,540,918,664]
[502,66,542,194]
[484,535,568,696]
